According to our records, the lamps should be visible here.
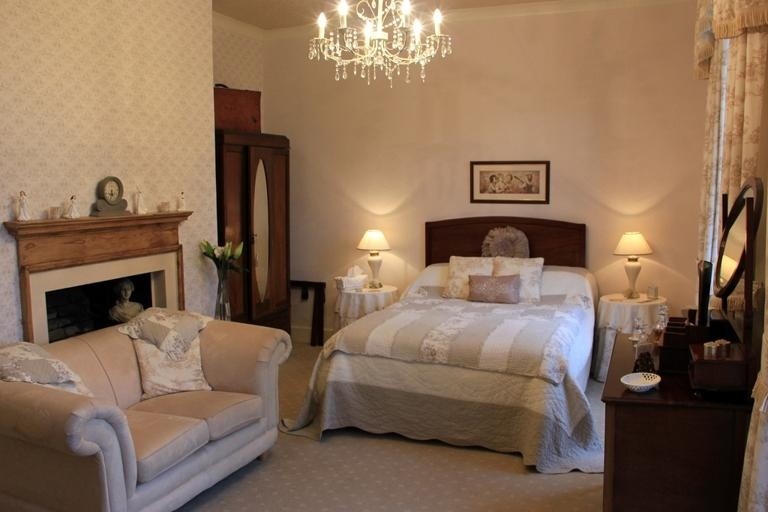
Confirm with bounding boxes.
[356,229,391,289]
[612,231,653,298]
[308,0,452,88]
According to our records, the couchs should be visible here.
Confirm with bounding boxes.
[0,309,293,512]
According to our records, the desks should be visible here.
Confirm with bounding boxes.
[600,318,760,512]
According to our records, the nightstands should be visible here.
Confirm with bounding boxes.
[333,285,398,330]
[591,293,667,384]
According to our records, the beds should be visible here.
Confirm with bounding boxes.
[279,216,605,475]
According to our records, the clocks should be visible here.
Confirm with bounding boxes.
[88,176,131,218]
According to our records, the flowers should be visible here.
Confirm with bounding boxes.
[200,238,245,280]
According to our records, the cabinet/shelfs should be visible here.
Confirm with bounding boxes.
[217,129,292,335]
[3,209,194,345]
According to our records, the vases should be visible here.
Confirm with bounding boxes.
[215,281,231,321]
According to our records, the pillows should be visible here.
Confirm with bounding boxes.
[492,256,544,303]
[441,256,495,298]
[132,338,213,402]
[466,274,520,304]
[0,341,82,384]
[117,306,213,362]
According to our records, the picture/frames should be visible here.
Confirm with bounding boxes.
[469,160,550,205]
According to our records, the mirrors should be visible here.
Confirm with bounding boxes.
[712,176,764,298]
[248,158,271,303]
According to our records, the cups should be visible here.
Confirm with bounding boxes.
[48,204,63,217]
[156,201,171,212]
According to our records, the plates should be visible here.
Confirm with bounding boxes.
[620,372,662,392]
